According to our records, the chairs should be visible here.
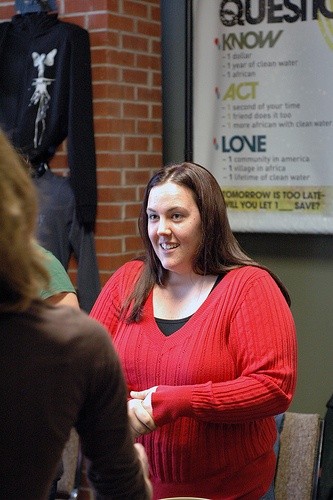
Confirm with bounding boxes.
[261,412,327,500]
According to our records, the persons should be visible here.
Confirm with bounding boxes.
[88,160,299,500]
[0,132,153,500]
[32,240,81,312]
